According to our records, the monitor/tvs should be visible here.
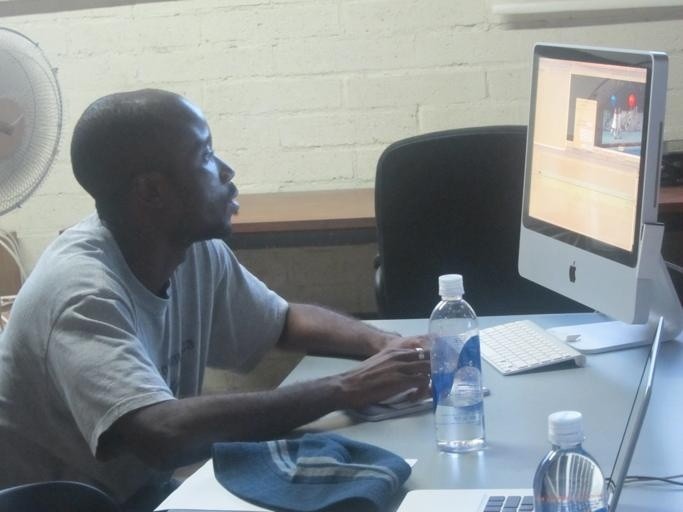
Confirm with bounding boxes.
[518,43,683,354]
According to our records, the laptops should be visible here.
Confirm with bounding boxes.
[398,317,664,512]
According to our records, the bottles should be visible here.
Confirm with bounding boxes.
[426,272,488,454]
[530,410,607,512]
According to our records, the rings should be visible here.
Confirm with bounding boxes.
[414,346,426,362]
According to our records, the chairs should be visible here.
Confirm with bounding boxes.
[0,476,125,511]
[368,120,600,313]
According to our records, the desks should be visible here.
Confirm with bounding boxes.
[220,175,682,258]
[148,313,683,512]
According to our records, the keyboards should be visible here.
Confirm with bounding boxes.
[456,319,586,375]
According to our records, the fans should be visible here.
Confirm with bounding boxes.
[1,24,65,221]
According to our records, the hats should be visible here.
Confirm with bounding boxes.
[211,433,411,511]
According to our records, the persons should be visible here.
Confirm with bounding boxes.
[1,88,440,510]
